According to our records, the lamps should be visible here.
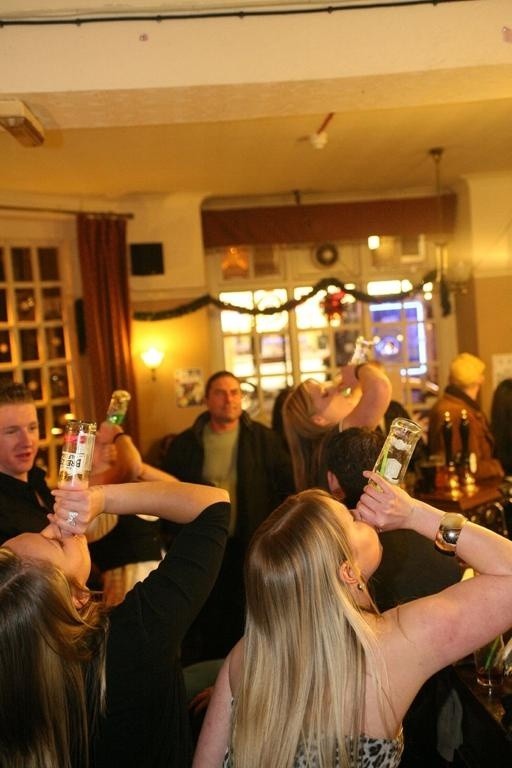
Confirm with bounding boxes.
[0,101,45,147]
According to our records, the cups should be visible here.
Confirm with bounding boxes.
[55,419,98,491]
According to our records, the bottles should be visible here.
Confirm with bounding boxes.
[475,636,505,690]
[368,416,424,492]
[106,390,132,427]
[342,339,369,396]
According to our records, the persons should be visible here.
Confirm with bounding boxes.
[0,351,512,767]
